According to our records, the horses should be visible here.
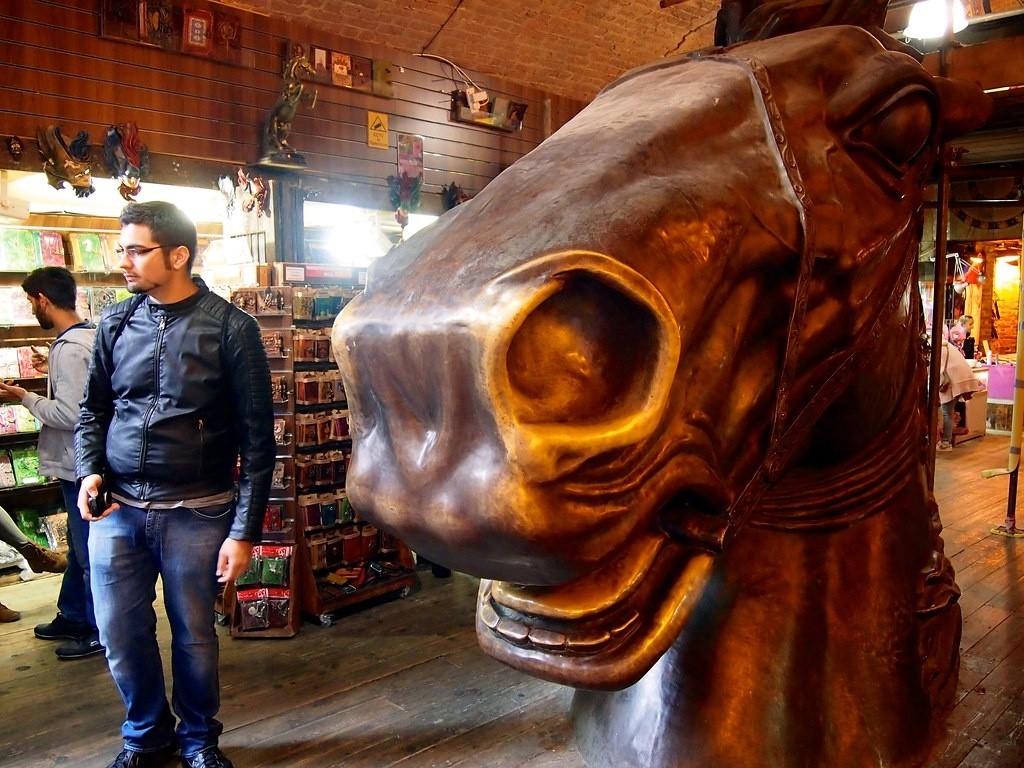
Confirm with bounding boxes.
[327,0,1023,767]
[260,53,318,154]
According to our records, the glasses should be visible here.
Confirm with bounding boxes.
[114,244,182,260]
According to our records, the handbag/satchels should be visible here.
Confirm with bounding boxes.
[936,372,950,393]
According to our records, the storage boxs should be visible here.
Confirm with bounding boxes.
[937,391,989,445]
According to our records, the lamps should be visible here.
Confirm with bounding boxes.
[946,252,969,295]
[957,258,988,283]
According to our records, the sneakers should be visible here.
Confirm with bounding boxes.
[19,539,70,575]
[53,629,107,660]
[936,439,952,452]
[102,738,185,768]
[0,602,20,624]
[32,614,91,642]
[178,745,234,768]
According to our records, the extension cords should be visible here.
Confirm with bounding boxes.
[466,88,480,114]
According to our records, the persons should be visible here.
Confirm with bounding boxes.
[0,264,106,658]
[958,314,976,359]
[74,202,280,768]
[923,324,978,452]
[949,325,966,358]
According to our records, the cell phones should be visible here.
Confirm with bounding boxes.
[88,488,113,518]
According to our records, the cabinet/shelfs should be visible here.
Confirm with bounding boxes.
[1,206,226,587]
[213,261,423,642]
